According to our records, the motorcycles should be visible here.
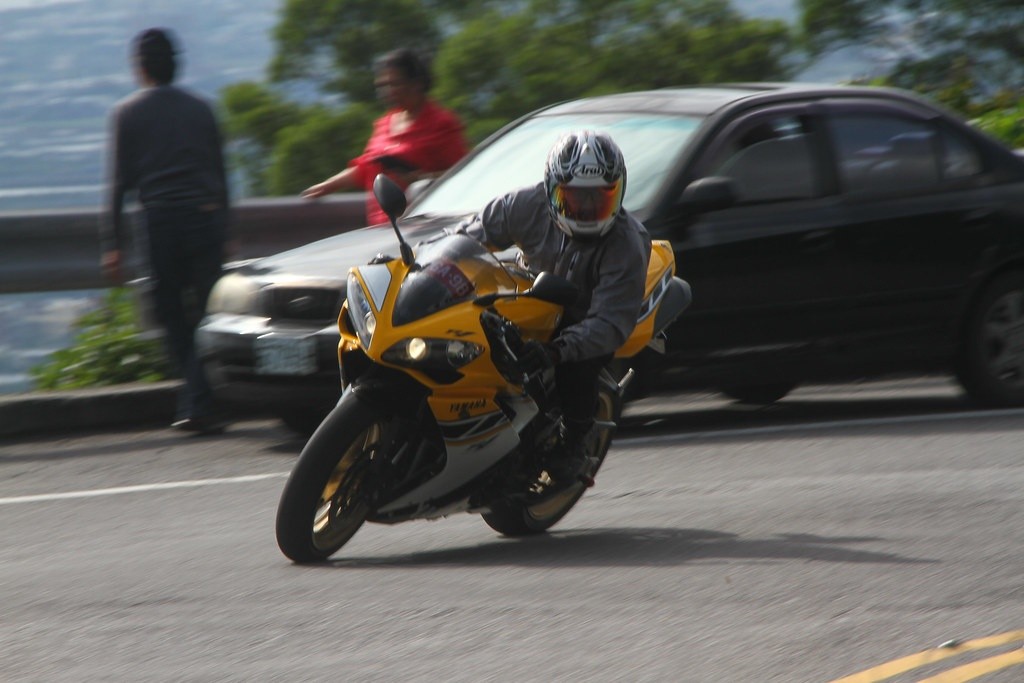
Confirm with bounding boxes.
[271,171,694,561]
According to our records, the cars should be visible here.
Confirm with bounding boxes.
[188,79,1024,447]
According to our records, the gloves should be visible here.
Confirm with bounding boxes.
[517,338,560,381]
[368,252,394,265]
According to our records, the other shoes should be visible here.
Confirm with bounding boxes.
[527,442,589,495]
[169,415,235,437]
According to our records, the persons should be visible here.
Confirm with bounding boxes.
[301,46,471,227]
[404,129,653,487]
[96,26,232,434]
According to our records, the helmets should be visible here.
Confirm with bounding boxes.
[544,131,627,237]
